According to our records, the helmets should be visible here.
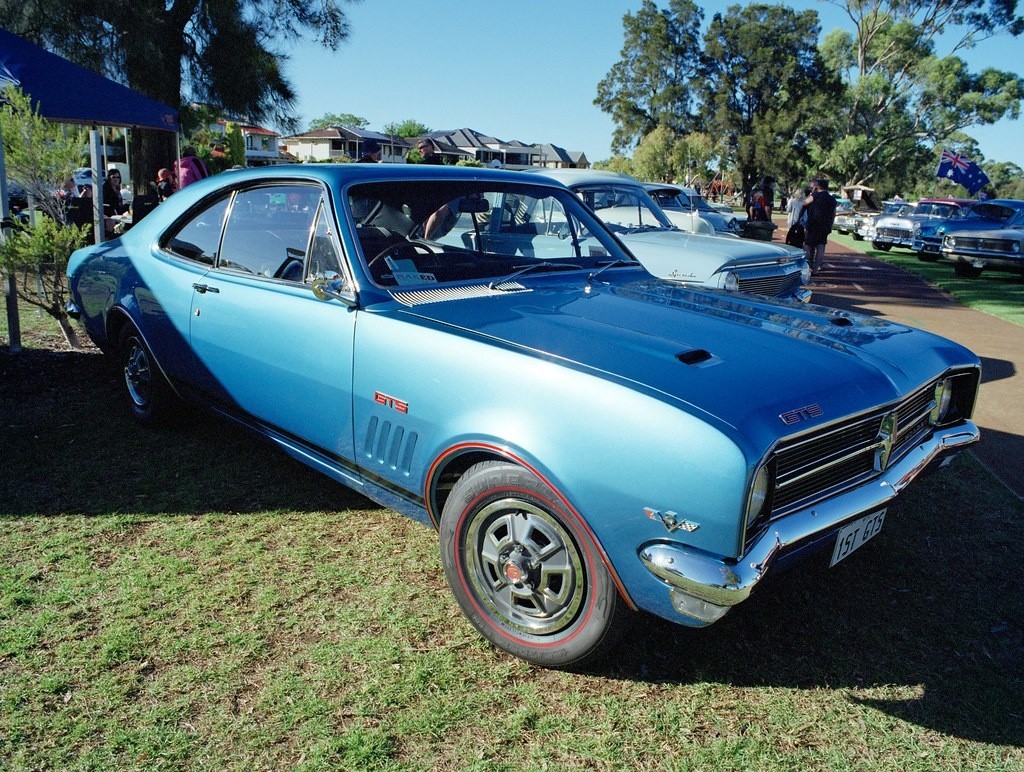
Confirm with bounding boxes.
[418,139,434,149]
[758,176,776,191]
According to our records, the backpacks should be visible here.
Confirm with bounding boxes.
[785,206,807,249]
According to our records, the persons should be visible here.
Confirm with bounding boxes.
[416,193,465,241]
[745,175,780,222]
[784,176,838,277]
[704,187,718,203]
[157,168,177,205]
[102,168,131,240]
[53,172,92,198]
[354,138,383,163]
[173,145,209,190]
[415,138,445,165]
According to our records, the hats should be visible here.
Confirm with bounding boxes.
[158,168,172,182]
[360,140,382,157]
[816,178,829,190]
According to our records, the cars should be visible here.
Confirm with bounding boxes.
[72,167,92,185]
[828,192,917,252]
[65,162,984,667]
[939,216,1024,279]
[681,188,749,233]
[405,167,813,303]
[533,181,713,236]
[910,198,1024,262]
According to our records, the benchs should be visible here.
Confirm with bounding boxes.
[193,225,415,275]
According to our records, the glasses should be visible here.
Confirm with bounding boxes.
[112,176,120,179]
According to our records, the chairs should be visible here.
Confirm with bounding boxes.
[66,196,94,246]
[110,195,158,239]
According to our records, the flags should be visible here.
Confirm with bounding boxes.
[936,148,991,198]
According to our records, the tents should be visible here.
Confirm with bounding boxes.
[0,29,182,352]
